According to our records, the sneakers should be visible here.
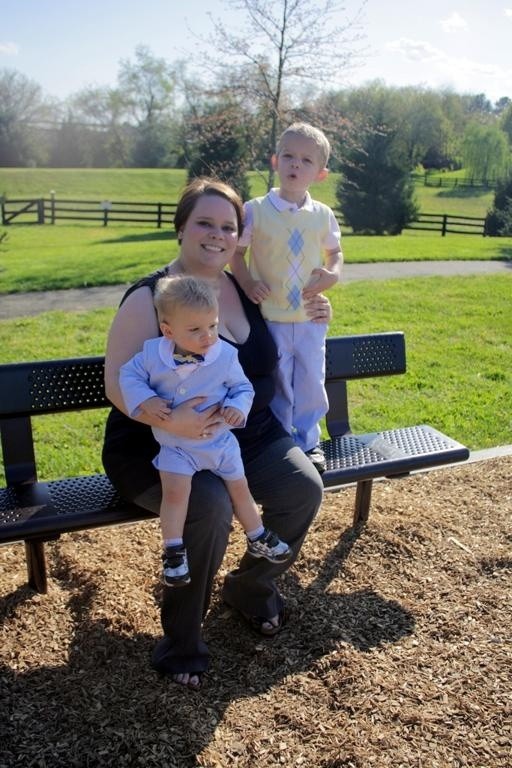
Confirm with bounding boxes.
[161,543,192,588]
[246,529,294,565]
[305,446,327,473]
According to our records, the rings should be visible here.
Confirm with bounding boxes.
[202,431,208,438]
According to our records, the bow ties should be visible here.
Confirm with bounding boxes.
[173,353,205,366]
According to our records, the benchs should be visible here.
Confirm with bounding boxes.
[1,332,469,595]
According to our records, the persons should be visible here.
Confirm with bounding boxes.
[101,171,334,693]
[225,120,346,474]
[115,272,296,589]
